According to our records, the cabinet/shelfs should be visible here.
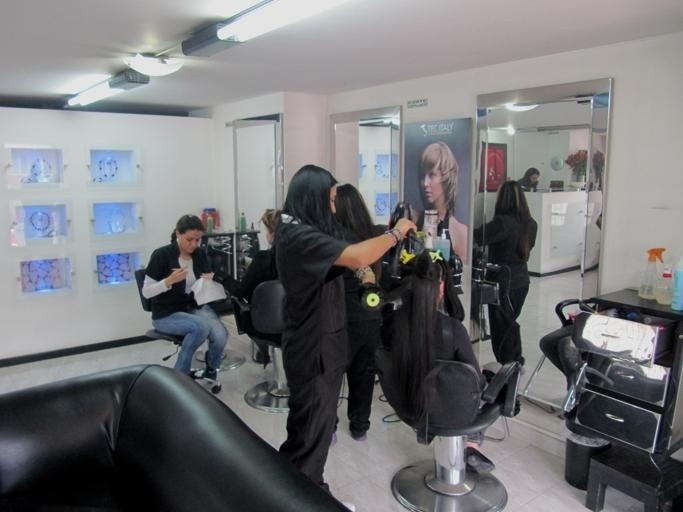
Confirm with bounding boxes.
[237,231,259,282]
[569,291,683,455]
[202,231,233,276]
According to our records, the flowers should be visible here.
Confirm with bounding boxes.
[564,150,587,174]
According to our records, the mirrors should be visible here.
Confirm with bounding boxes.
[232,112,285,281]
[468,77,613,409]
[328,106,406,227]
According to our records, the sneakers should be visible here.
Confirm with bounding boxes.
[205,350,217,385]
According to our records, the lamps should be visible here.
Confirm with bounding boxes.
[182,0,348,58]
[122,53,183,75]
[67,69,150,108]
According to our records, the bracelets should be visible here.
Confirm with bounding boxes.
[385,229,401,246]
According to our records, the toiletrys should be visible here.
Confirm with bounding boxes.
[434,228,451,263]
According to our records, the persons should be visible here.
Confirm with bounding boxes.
[325,184,385,443]
[474,179,538,371]
[414,141,467,264]
[200,208,282,370]
[141,216,227,392]
[359,251,497,476]
[275,166,418,512]
[516,169,540,191]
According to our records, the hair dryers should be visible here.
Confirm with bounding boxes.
[384,200,416,276]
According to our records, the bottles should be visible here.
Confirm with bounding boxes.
[654,249,682,312]
[239,211,245,233]
[205,213,213,234]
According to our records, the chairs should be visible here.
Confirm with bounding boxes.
[135,269,221,394]
[231,280,291,413]
[373,345,520,512]
[190,269,245,371]
[525,299,596,392]
[0,365,352,512]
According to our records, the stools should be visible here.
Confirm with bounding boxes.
[585,448,683,512]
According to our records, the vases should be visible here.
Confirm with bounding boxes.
[577,171,585,183]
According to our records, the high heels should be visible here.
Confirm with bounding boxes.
[255,350,271,368]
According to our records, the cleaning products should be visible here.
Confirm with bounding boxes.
[207,215,213,234]
[637,246,682,312]
[240,211,246,233]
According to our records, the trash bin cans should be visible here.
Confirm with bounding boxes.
[564,435,611,490]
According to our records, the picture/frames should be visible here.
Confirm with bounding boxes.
[479,143,507,192]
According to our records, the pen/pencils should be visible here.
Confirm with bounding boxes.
[172,268,190,270]
